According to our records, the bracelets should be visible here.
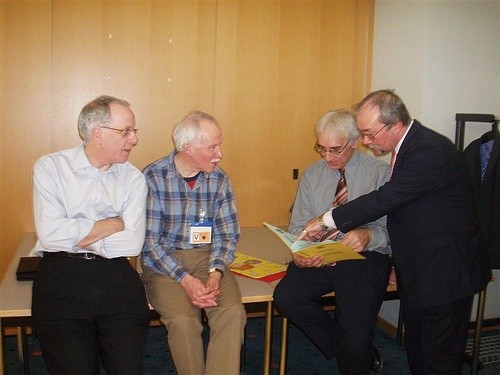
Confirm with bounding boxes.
[208,268,224,279]
[320,214,330,232]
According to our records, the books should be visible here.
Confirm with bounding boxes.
[263,222,367,266]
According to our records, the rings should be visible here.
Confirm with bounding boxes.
[302,229,307,232]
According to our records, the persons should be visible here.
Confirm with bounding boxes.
[295,89,492,375]
[32,96,148,375]
[139,110,247,375]
[274,110,393,375]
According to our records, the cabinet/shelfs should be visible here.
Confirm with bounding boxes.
[0,0,377,335]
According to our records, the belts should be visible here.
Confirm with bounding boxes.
[43,250,101,260]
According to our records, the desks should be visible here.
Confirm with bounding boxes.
[0,227,495,375]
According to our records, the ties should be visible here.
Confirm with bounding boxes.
[320,169,349,242]
[390,151,396,175]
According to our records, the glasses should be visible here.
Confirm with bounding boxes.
[100,125,138,137]
[360,122,389,141]
[314,138,351,155]
